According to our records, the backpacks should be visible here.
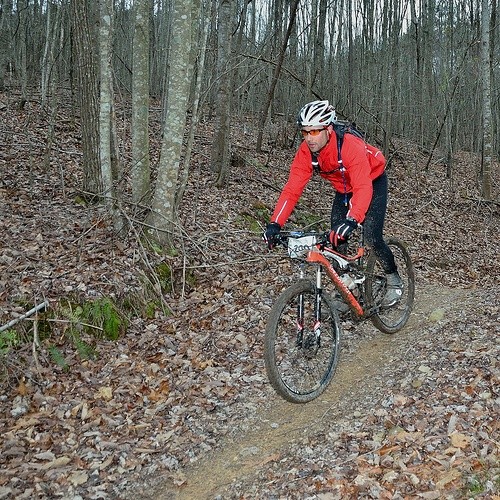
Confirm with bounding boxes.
[311,120,363,206]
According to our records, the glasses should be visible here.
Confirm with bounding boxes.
[301,128,328,136]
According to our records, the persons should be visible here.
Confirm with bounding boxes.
[263,100,405,308]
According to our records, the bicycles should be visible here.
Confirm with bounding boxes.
[257,218,417,404]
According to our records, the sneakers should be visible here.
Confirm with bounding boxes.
[382,271,404,306]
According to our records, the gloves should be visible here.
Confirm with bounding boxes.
[264,223,282,250]
[330,219,358,246]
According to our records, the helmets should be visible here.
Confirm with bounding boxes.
[297,100,337,126]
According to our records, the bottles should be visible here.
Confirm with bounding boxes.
[340,274,364,303]
